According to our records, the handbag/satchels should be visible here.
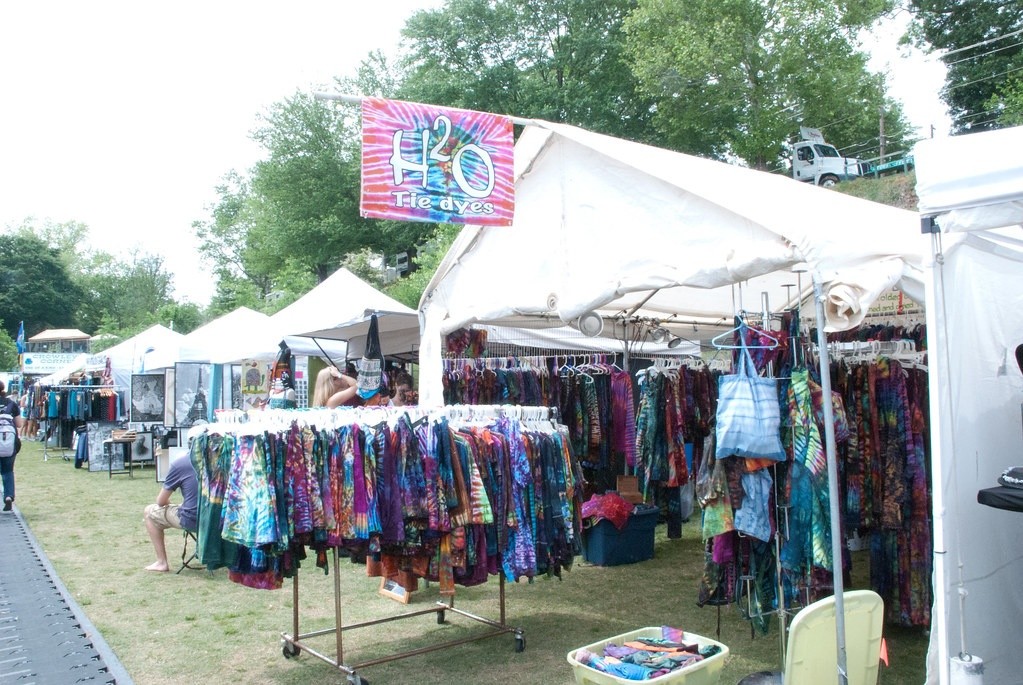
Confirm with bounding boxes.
[809,380,850,443]
[717,324,785,462]
[356,315,385,398]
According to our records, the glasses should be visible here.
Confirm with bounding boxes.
[332,377,337,380]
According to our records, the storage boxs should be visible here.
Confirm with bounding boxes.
[566,626,728,685]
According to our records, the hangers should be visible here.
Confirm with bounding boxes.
[636,356,731,380]
[807,339,928,374]
[43,384,119,398]
[858,308,926,334]
[712,309,778,350]
[444,352,622,384]
[189,404,564,434]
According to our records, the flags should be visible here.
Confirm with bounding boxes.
[341,98,525,228]
[16,323,26,354]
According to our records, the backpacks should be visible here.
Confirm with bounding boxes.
[0,400,16,457]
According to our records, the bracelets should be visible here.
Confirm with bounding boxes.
[338,373,342,379]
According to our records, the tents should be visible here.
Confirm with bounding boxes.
[415,120,936,685]
[35,352,102,384]
[87,306,282,427]
[913,124,1023,685]
[271,264,423,410]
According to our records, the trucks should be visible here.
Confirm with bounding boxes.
[792,127,872,189]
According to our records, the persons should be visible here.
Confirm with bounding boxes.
[311,364,357,409]
[141,435,199,573]
[0,376,22,515]
[20,388,41,443]
[386,369,419,408]
[7,387,19,403]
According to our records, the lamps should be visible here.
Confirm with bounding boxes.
[667,333,681,348]
[648,328,666,343]
[577,311,604,338]
